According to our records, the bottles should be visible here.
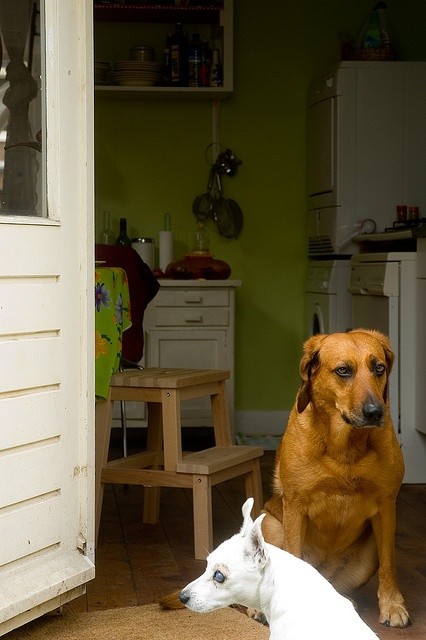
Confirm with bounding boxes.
[191,223,211,256]
[99,210,114,245]
[410,205,419,221]
[397,205,406,221]
[188,49,199,86]
[116,217,131,245]
[199,38,211,86]
[163,48,171,74]
[171,43,181,85]
[212,50,222,86]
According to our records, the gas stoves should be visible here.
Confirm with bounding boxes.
[365,217,426,235]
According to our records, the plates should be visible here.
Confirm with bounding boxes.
[113,61,163,85]
[95,61,111,84]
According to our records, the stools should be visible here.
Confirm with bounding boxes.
[95,366,264,560]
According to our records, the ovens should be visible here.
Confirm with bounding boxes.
[349,253,425,453]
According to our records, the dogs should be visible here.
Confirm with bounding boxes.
[177,496,382,640]
[156,326,413,630]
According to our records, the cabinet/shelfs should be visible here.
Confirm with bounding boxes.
[112,279,243,444]
[94,0,233,103]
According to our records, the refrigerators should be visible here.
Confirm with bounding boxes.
[306,61,426,258]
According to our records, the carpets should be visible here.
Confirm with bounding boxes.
[1,600,270,640]
